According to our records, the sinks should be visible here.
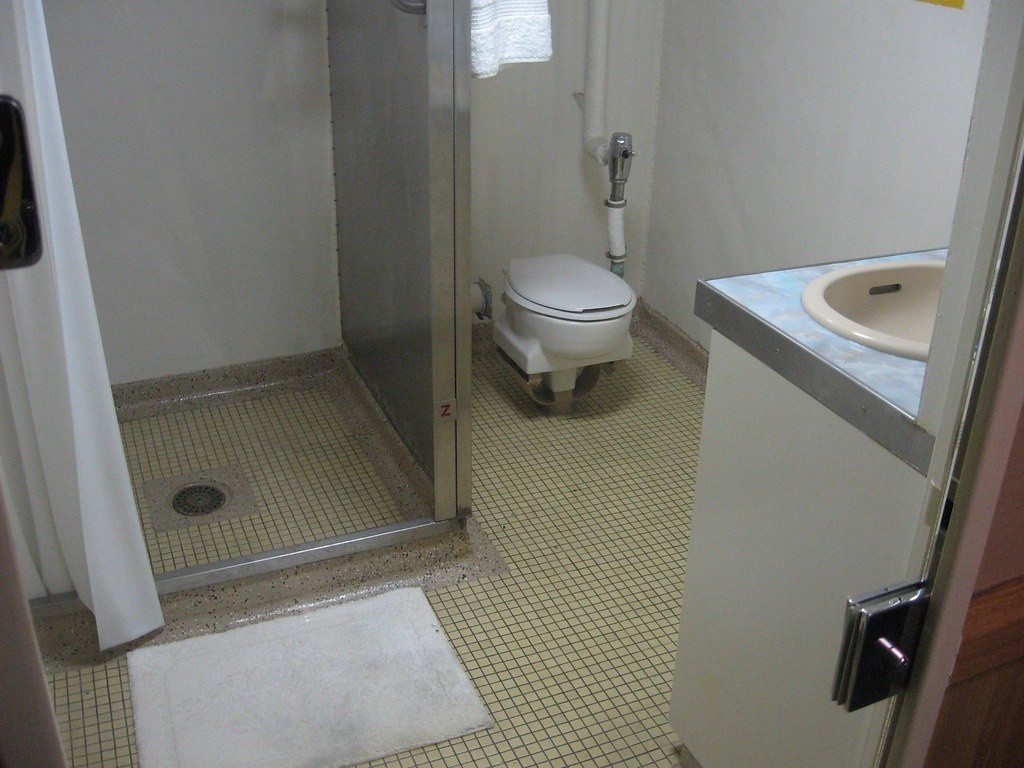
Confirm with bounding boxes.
[802,255,949,361]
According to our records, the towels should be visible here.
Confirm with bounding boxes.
[469,0,553,81]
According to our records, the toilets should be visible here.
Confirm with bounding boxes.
[491,249,635,376]
